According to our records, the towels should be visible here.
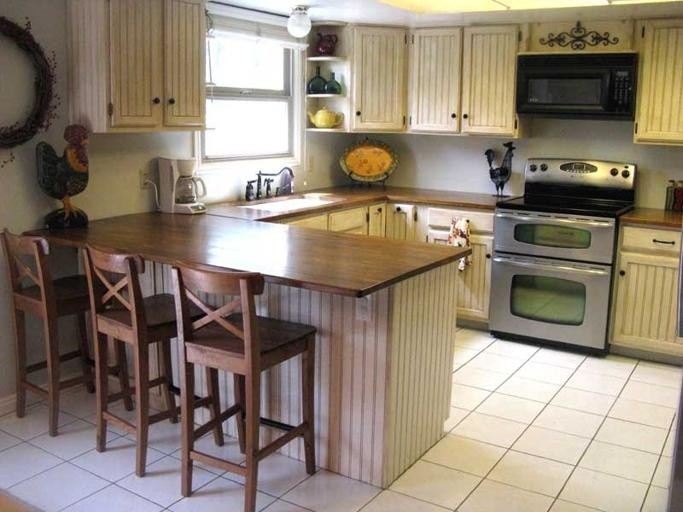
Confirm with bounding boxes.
[446,217,473,272]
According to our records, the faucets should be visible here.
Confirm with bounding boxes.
[258,166,296,199]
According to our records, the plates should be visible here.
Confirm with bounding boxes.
[336,138,398,182]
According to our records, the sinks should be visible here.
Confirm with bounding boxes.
[234,194,348,212]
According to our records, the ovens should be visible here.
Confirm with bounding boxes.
[487,211,617,355]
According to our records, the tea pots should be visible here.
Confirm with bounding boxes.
[315,32,337,56]
[306,108,343,129]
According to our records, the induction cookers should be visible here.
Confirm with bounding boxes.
[505,195,635,217]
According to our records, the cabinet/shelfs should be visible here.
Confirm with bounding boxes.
[65,0,207,134]
[426,206,495,324]
[269,201,417,240]
[633,18,683,148]
[350,25,404,133]
[406,23,532,138]
[303,21,350,133]
[607,223,682,358]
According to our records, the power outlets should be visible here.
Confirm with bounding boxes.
[139,169,155,189]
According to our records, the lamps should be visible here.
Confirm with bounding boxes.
[286,1,312,39]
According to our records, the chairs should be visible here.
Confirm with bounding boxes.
[79,239,224,477]
[170,258,318,511]
[1,226,133,436]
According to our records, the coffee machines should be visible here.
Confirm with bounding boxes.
[155,155,206,214]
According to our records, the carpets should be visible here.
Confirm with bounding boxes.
[0,489,45,512]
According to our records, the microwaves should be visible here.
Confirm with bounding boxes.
[513,52,636,121]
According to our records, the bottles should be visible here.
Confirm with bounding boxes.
[323,72,340,95]
[281,171,293,194]
[308,66,327,94]
[662,179,683,211]
